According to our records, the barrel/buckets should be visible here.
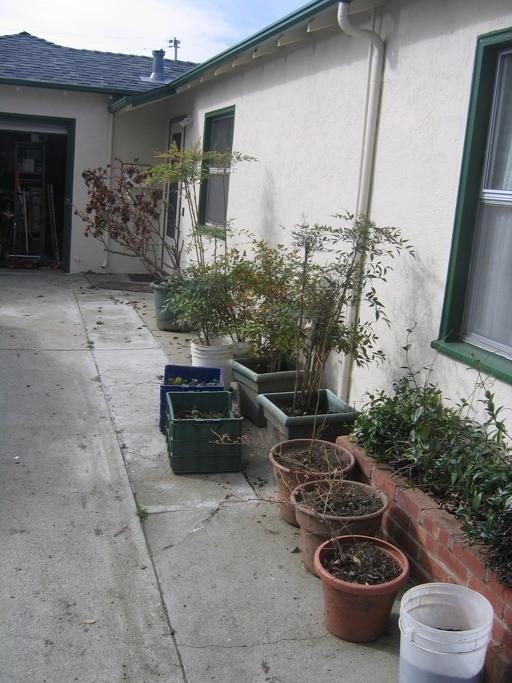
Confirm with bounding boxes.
[396,579,494,683]
[191,334,237,390]
[234,340,253,357]
[150,277,195,333]
